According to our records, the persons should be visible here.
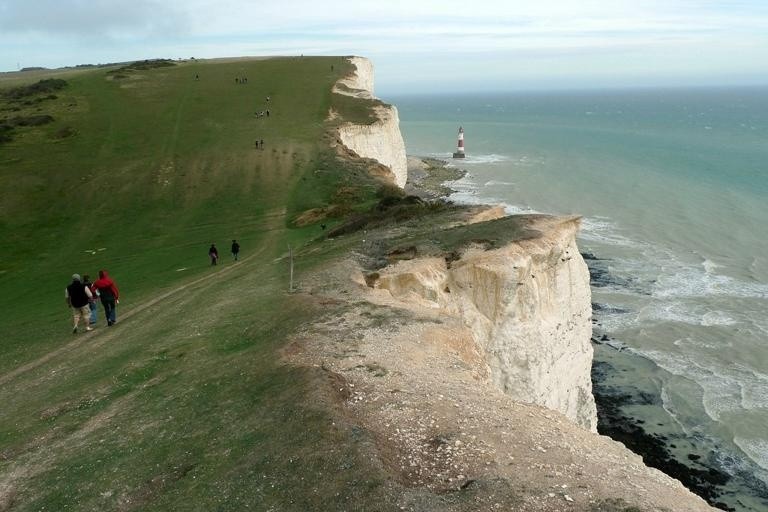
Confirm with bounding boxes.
[209,244,218,265]
[256,138,263,149]
[90,270,120,326]
[65,273,94,333]
[231,239,240,260]
[253,108,270,119]
[267,95,270,102]
[81,275,100,324]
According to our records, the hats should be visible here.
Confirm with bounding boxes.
[72,274,80,281]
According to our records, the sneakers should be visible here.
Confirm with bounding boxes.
[72,324,78,333]
[85,326,93,332]
[108,319,112,325]
